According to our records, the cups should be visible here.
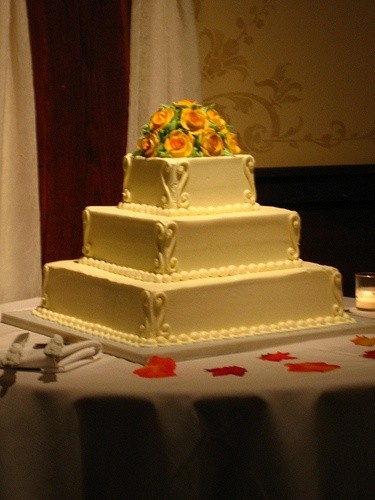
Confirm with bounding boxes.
[355,272,375,310]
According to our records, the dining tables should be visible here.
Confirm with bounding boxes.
[0,295,375,500]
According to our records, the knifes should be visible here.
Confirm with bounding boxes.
[7,332,29,364]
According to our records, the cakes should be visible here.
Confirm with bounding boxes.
[32,151,356,348]
